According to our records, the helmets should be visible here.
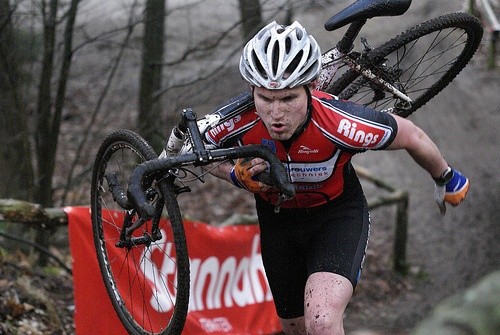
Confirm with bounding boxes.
[238,20,324,89]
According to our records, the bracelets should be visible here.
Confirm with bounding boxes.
[433,164,454,185]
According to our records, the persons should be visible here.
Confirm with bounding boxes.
[186,20,471,334]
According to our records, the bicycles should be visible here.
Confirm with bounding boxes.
[87,0,484,335]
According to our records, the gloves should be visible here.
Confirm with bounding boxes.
[435,169,470,217]
[230,143,268,194]
